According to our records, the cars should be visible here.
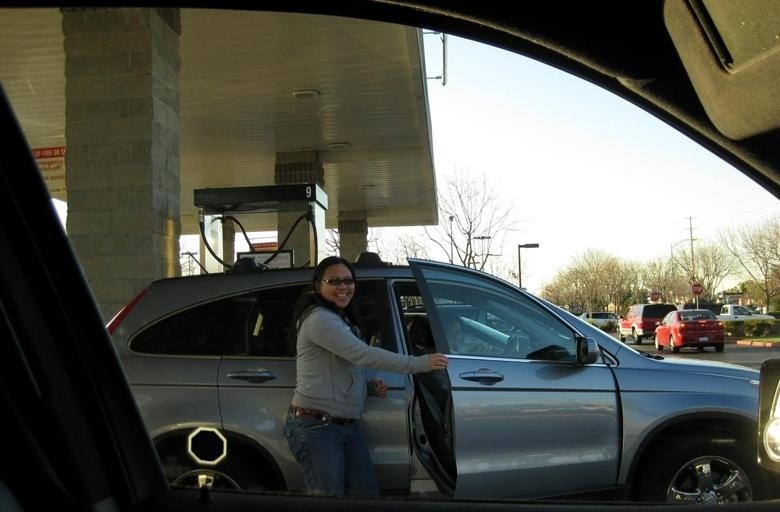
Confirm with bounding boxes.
[104,255,779,508]
[580,312,620,332]
[654,309,724,353]
[617,304,679,345]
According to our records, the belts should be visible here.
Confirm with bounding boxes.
[287,404,355,427]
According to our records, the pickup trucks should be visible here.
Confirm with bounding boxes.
[714,304,776,322]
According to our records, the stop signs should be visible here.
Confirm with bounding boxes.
[650,292,659,301]
[692,283,703,295]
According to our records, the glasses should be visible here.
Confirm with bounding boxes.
[321,276,355,285]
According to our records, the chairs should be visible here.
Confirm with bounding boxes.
[406,316,435,355]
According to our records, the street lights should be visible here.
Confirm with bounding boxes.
[518,243,539,288]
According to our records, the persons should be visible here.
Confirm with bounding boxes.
[283,255,450,498]
[419,312,463,409]
